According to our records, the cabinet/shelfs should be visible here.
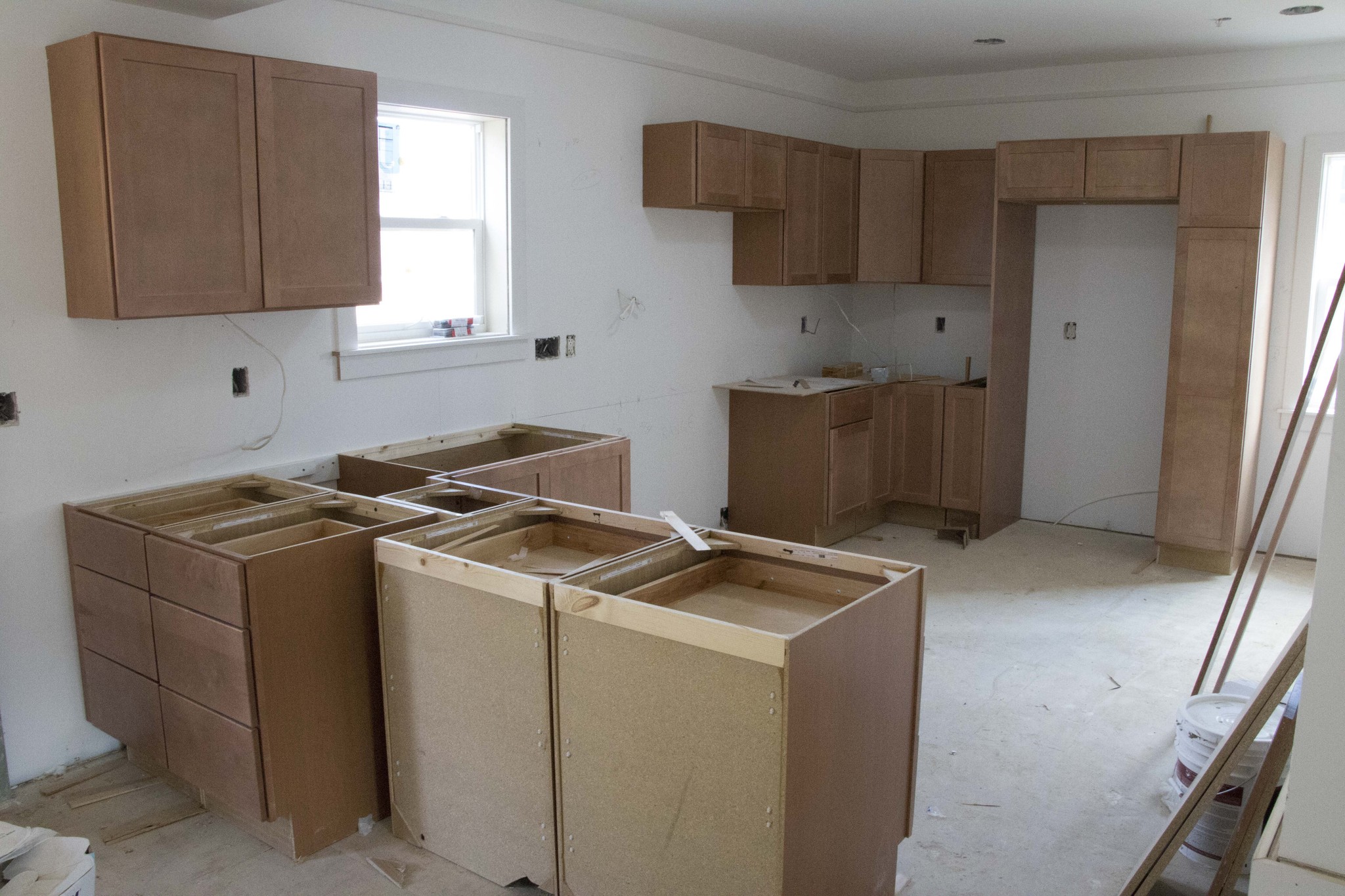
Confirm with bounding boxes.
[996,135,1182,205]
[733,137,860,285]
[64,506,270,825]
[45,30,382,320]
[858,148,922,284]
[452,438,631,514]
[643,120,787,213]
[1156,132,1285,549]
[924,148,995,287]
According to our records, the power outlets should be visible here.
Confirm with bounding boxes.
[566,338,574,355]
[1066,325,1076,338]
[828,383,892,524]
[894,383,984,511]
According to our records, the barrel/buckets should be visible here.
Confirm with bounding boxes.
[1167,693,1287,874]
